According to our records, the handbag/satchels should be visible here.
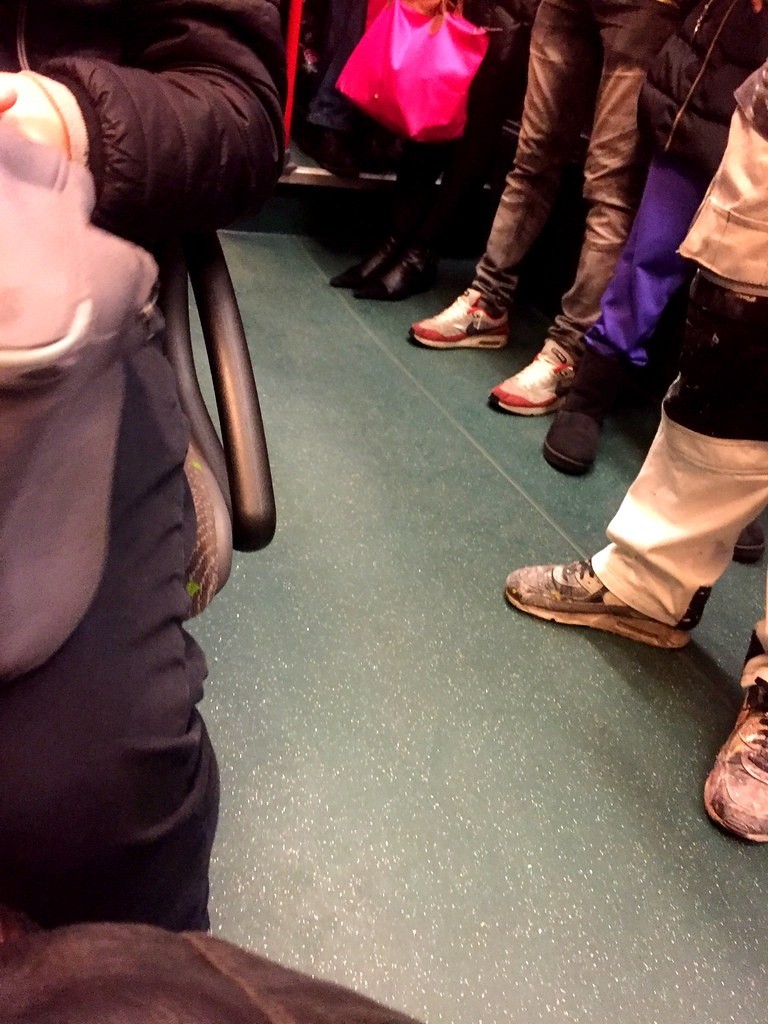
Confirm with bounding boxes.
[0,112,158,683]
[334,0,489,143]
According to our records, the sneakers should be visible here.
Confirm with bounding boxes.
[488,338,577,417]
[505,559,691,649]
[704,677,768,843]
[409,288,511,349]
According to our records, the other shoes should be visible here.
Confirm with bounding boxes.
[342,113,408,173]
[294,122,362,174]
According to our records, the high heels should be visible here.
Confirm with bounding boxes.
[353,244,438,301]
[330,236,408,289]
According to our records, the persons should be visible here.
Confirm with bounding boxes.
[329,1,536,301]
[407,0,672,417]
[542,0,768,555]
[0,0,315,931]
[1,925,424,1024]
[503,62,768,844]
[296,1,368,179]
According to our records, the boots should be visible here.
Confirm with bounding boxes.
[543,349,625,475]
[731,519,765,563]
